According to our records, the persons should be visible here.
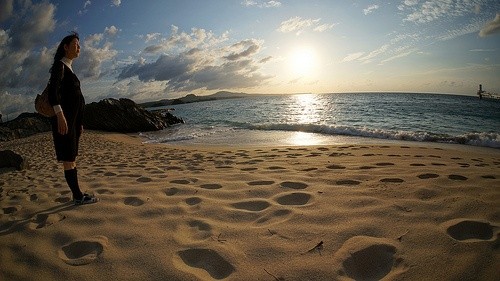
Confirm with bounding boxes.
[46,30,99,204]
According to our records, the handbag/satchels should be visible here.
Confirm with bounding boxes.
[35,64,64,118]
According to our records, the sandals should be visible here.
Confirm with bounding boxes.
[74,192,99,205]
